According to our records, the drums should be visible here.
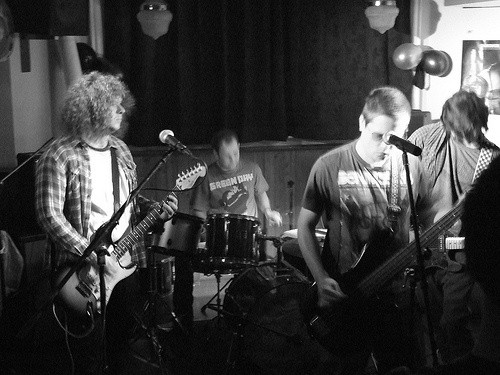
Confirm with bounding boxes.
[204,210,260,275]
[224,260,323,374]
[132,253,174,299]
[148,207,204,260]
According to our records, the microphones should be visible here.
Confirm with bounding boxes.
[159,129,198,160]
[382,131,422,156]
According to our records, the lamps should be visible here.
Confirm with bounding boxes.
[136,0,173,39]
[364,0,400,34]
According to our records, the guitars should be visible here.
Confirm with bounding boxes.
[407,222,466,274]
[53,163,207,318]
[235,191,478,375]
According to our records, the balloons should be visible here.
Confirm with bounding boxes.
[392,43,450,77]
[463,73,489,99]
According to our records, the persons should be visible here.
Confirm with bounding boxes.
[296,86,500,375]
[172,128,286,332]
[35,70,179,375]
